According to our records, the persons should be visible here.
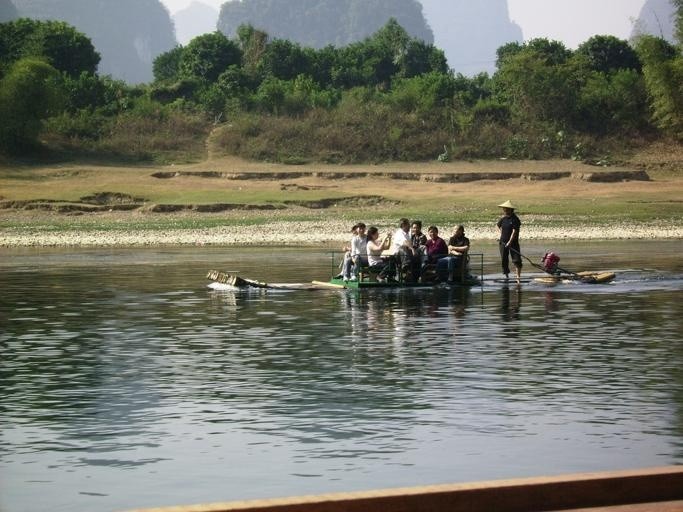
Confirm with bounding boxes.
[343,287,468,364]
[495,200,522,283]
[335,217,478,284]
[499,285,524,371]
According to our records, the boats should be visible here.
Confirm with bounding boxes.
[205,255,618,291]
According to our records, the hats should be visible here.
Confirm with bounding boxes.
[497,201,517,209]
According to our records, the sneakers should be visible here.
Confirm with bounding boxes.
[376,275,385,282]
[334,275,357,282]
